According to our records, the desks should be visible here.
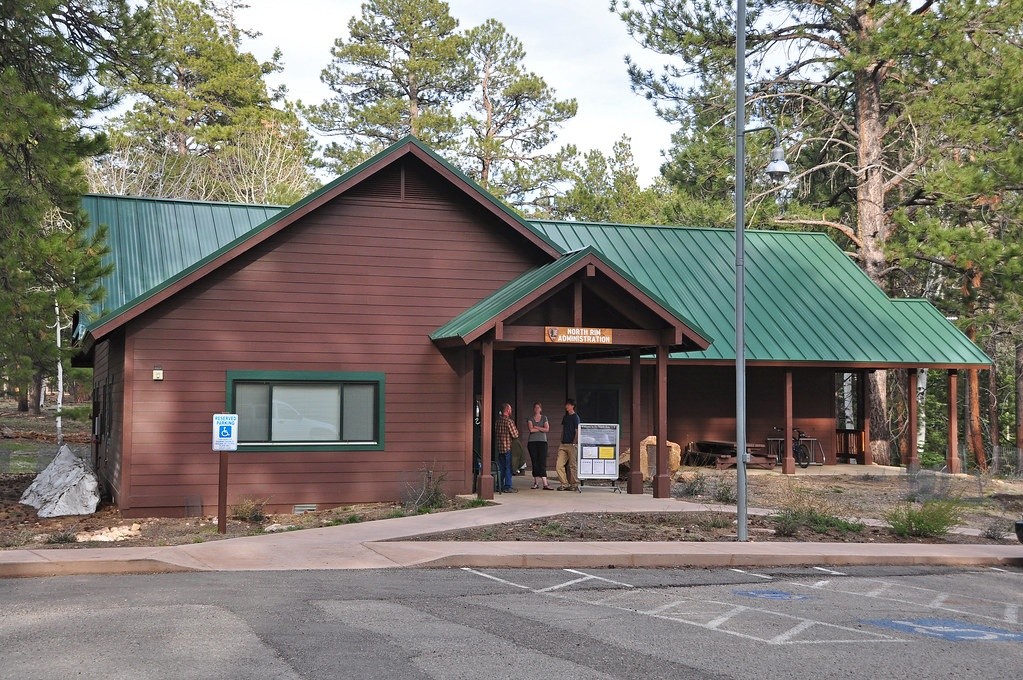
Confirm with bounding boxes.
[697,439,768,469]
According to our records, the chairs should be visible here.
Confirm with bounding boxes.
[473,448,502,495]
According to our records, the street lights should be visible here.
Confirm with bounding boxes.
[734,123,789,542]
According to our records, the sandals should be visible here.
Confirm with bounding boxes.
[530,483,539,489]
[543,484,554,490]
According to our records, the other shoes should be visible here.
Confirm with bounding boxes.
[567,486,578,491]
[557,485,569,491]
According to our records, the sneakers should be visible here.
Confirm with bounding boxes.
[496,485,504,492]
[504,487,518,493]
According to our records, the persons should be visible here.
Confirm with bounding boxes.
[494,403,519,493]
[527,402,554,490]
[555,399,580,491]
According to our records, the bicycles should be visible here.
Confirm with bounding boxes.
[773,425,811,469]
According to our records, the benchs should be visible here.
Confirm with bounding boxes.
[732,451,779,470]
[687,450,731,470]
